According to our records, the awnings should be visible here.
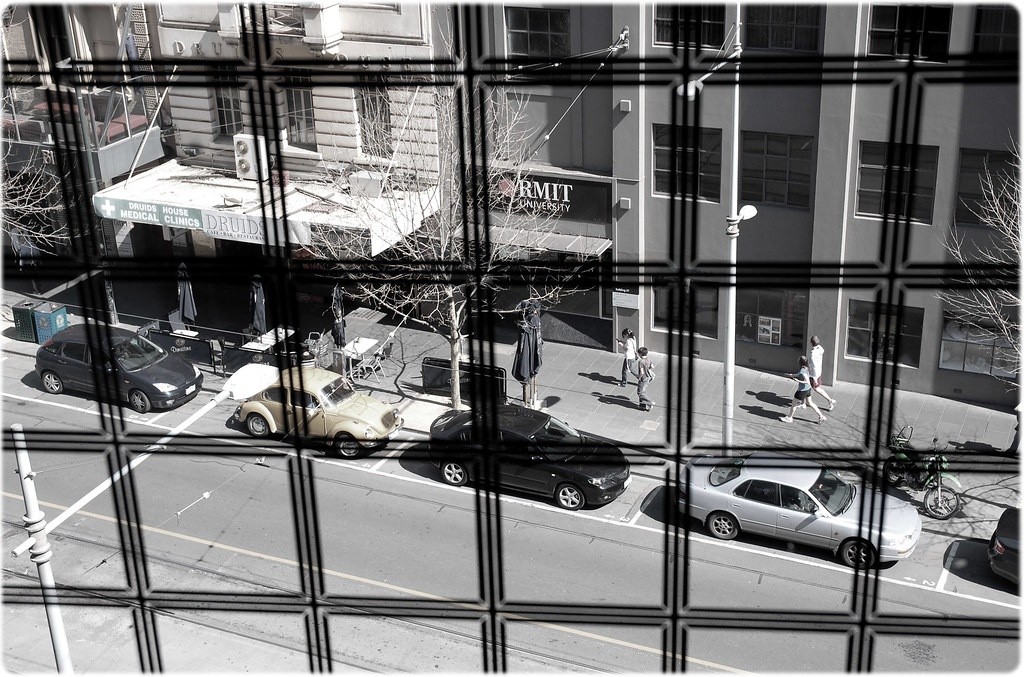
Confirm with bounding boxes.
[91,158,441,257]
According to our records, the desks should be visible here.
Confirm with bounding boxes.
[341,337,375,381]
[173,329,199,339]
[242,327,295,356]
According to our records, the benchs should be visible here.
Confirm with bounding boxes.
[137,319,160,337]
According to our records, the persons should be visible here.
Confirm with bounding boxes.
[17,230,42,293]
[11,222,28,267]
[796,335,837,411]
[778,355,827,424]
[1004,402,1021,456]
[629,346,656,413]
[617,327,641,388]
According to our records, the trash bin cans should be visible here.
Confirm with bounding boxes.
[12,299,44,343]
[32,302,68,345]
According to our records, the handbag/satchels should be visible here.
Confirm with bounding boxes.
[630,358,656,383]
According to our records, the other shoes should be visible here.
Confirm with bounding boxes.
[780,416,792,422]
[649,401,655,411]
[638,406,646,409]
[829,399,836,411]
[618,384,625,387]
[797,404,806,409]
[818,416,826,424]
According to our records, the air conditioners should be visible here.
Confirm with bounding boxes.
[233,133,269,181]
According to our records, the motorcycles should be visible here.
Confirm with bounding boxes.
[883,425,963,520]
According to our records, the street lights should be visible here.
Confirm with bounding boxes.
[677,4,758,456]
[10,363,281,674]
[721,202,759,456]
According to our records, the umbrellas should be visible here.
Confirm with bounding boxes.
[253,280,266,335]
[512,297,543,408]
[329,284,346,351]
[176,261,197,331]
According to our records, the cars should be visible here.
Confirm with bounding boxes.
[233,366,404,460]
[668,450,923,569]
[35,323,204,414]
[428,404,632,512]
[986,507,1020,584]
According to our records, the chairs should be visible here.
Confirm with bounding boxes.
[168,309,186,332]
[204,336,223,374]
[357,351,386,383]
[313,340,331,368]
